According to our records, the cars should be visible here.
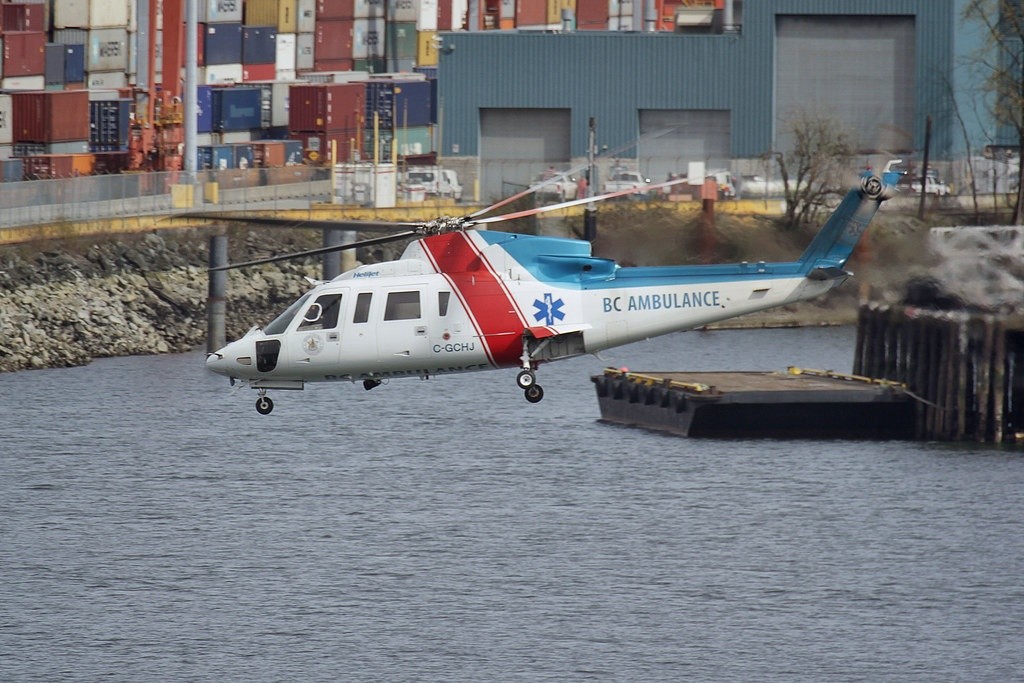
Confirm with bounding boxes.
[911,175,950,196]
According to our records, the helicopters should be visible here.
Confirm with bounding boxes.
[154,122,908,416]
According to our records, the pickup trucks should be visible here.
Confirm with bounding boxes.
[601,170,653,202]
[530,170,580,205]
